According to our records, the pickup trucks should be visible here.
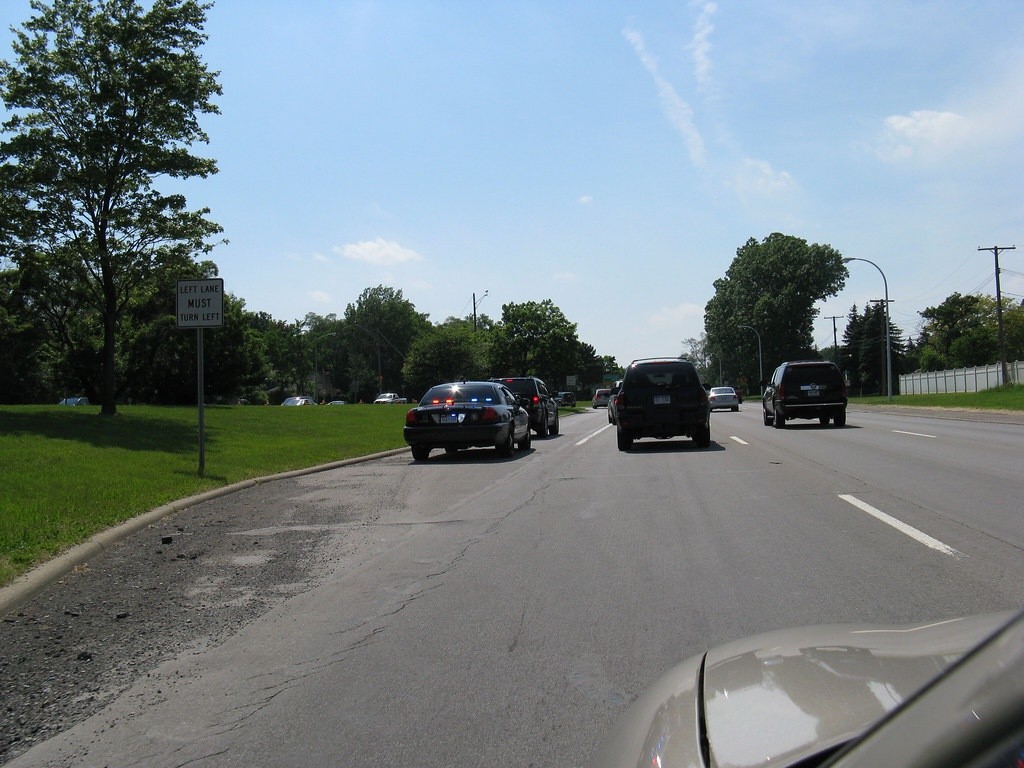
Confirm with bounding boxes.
[374,393,407,405]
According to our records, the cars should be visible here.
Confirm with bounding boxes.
[404,383,532,461]
[592,390,614,408]
[281,397,317,406]
[706,387,739,412]
[555,391,577,407]
[606,380,624,424]
[58,397,90,406]
[735,390,743,404]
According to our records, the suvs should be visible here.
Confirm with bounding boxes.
[610,357,712,451]
[759,361,848,429]
[486,376,560,438]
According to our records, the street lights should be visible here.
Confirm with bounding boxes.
[738,325,764,401]
[315,333,336,402]
[840,257,894,401]
[472,292,488,334]
[710,353,722,387]
